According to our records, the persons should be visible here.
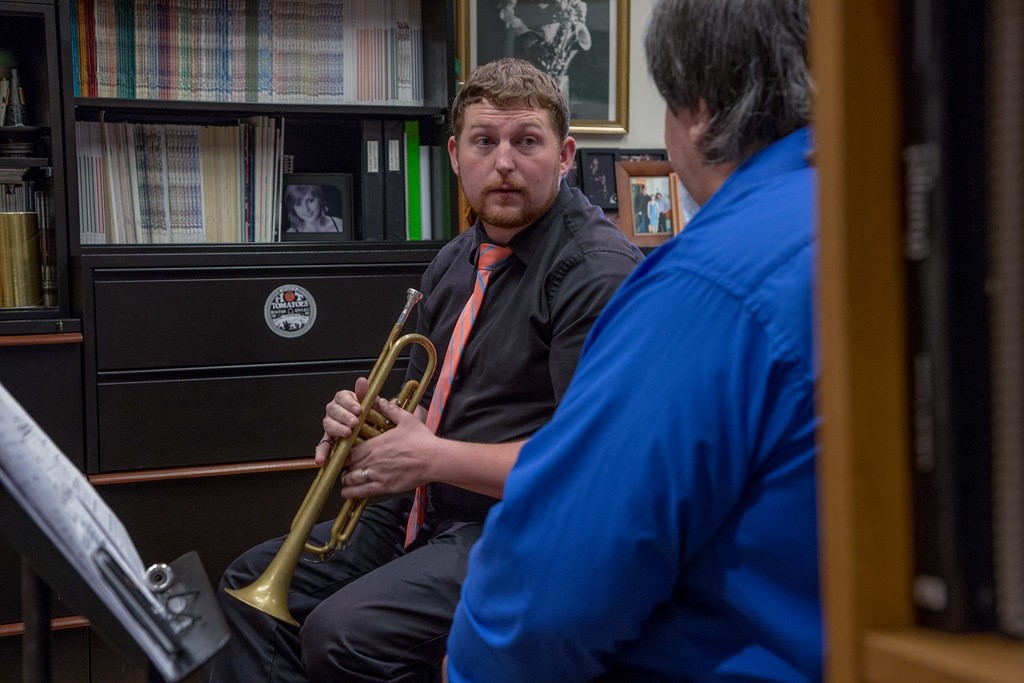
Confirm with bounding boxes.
[435,0,819,683]
[285,184,344,233]
[635,185,668,232]
[586,155,610,204]
[208,58,649,683]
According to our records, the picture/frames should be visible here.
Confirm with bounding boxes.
[576,146,701,248]
[275,172,354,241]
[457,0,631,134]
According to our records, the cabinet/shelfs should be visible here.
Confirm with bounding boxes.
[56,0,458,593]
[0,0,91,639]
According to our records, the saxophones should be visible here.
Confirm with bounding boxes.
[533,0,593,84]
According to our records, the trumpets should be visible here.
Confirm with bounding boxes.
[221,285,438,628]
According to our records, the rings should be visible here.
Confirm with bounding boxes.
[320,440,330,444]
[363,467,369,482]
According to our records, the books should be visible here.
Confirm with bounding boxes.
[68,0,423,244]
